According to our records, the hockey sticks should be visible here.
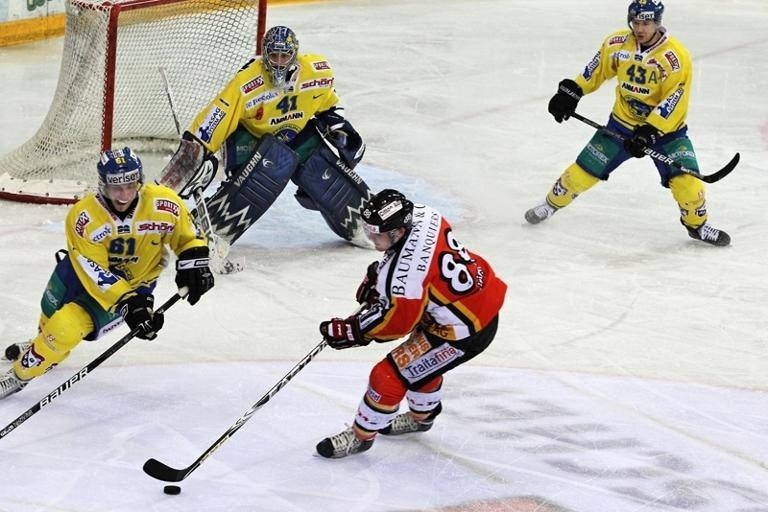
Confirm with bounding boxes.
[159,67,242,274]
[572,111,740,182]
[144,301,373,481]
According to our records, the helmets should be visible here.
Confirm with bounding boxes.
[360,189,414,231]
[628,0,664,22]
[97,147,144,198]
[261,26,299,88]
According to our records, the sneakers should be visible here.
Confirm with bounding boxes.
[378,411,433,435]
[0,367,29,400]
[525,203,556,224]
[6,342,33,362]
[680,216,730,246]
[317,426,374,458]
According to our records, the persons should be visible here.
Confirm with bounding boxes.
[515,0,734,248]
[158,27,378,256]
[0,148,216,402]
[315,189,507,458]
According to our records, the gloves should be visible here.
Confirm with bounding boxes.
[623,124,658,158]
[175,246,215,305]
[114,293,165,340]
[356,261,379,309]
[319,316,370,350]
[549,79,583,123]
[312,106,367,169]
